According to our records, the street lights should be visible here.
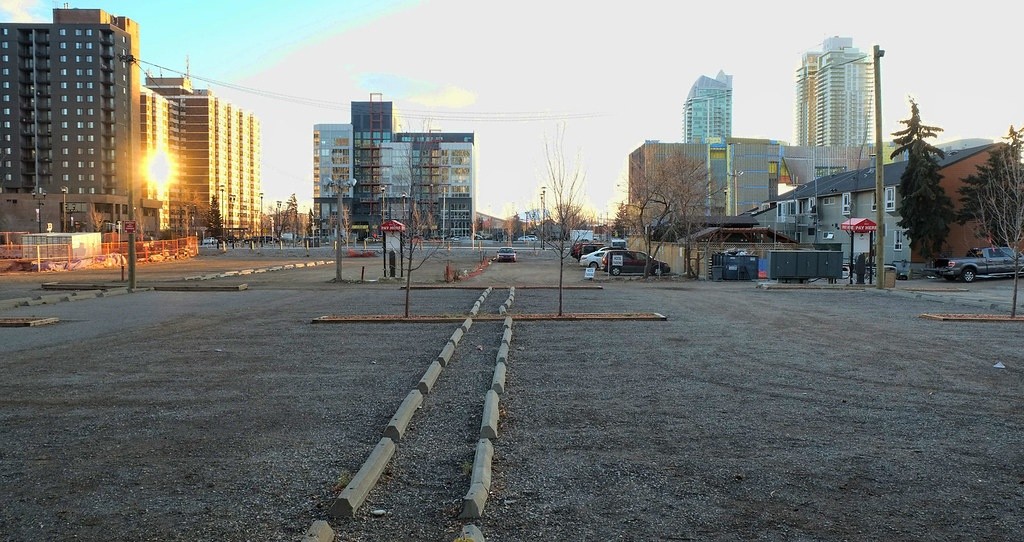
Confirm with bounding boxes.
[32,190,47,232]
[187,205,197,235]
[382,188,385,222]
[65,203,76,232]
[277,200,281,245]
[540,186,547,248]
[219,184,225,242]
[259,193,264,247]
[402,193,406,223]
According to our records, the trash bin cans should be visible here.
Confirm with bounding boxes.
[712,253,759,280]
[884,265,897,288]
[304,237,319,247]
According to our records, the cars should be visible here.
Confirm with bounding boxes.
[580,251,606,271]
[497,247,517,262]
[518,235,538,241]
[202,236,279,245]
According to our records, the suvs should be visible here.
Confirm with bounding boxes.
[570,239,627,262]
[601,250,670,276]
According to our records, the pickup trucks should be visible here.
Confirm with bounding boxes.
[934,247,1024,283]
